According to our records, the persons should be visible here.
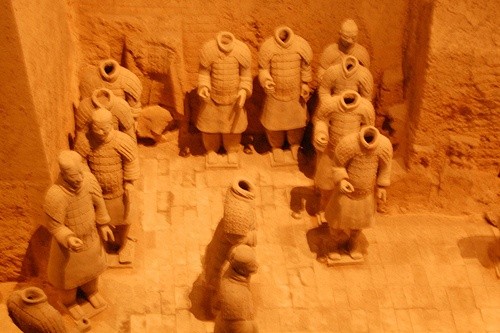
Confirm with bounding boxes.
[7,285,92,333]
[319,55,374,100]
[212,243,259,333]
[310,89,375,211]
[323,125,392,260]
[41,149,115,320]
[74,108,139,263]
[257,26,313,161]
[224,178,258,248]
[75,88,137,141]
[196,31,254,165]
[80,58,144,133]
[315,19,371,83]
